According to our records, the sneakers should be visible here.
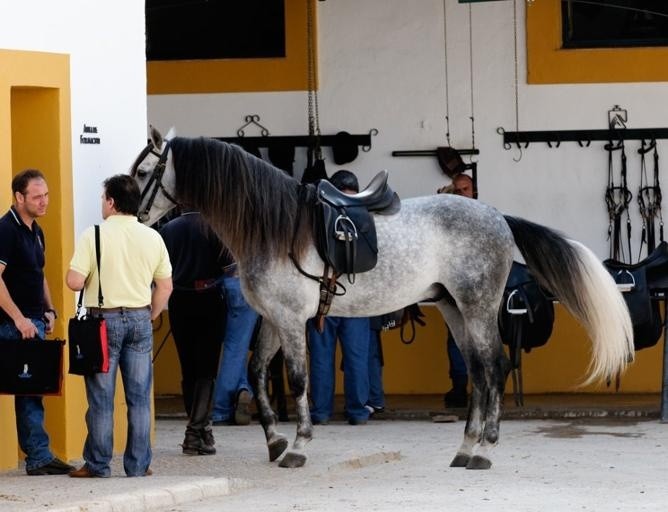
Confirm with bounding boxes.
[214,389,251,425]
[443,388,466,402]
[344,404,385,414]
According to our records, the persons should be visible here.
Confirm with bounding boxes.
[306,169,371,426]
[0,168,77,477]
[364,315,386,416]
[211,238,262,427]
[444,174,511,410]
[159,192,230,457]
[65,174,175,479]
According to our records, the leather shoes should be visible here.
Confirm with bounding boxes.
[70,465,99,477]
[143,468,153,475]
[25,458,76,476]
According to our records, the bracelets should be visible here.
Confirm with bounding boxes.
[44,307,57,323]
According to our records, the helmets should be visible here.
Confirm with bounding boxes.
[329,170,358,193]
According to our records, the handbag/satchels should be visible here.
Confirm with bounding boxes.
[68,318,110,376]
[0,334,65,396]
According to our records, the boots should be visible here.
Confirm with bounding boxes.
[181,379,217,454]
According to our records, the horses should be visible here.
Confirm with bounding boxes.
[130,122,636,470]
